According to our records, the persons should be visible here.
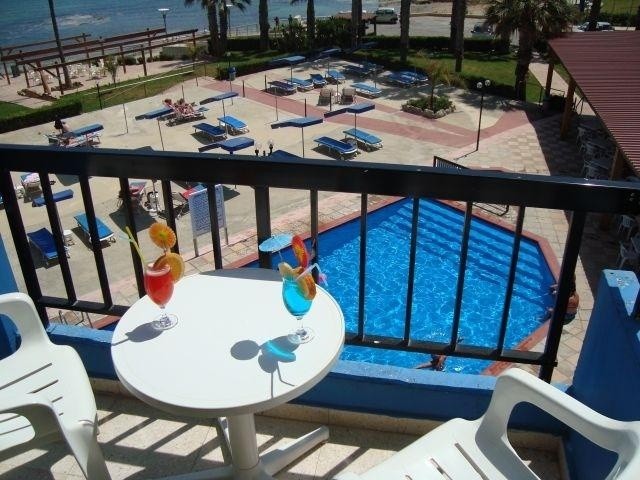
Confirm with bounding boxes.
[165,99,198,114]
[62,121,71,134]
[412,337,463,371]
[540,282,580,325]
[549,268,576,296]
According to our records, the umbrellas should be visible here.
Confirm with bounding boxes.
[258,234,295,261]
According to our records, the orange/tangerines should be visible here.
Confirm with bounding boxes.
[294,266,317,300]
[154,253,185,283]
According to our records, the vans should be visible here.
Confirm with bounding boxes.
[370,7,398,24]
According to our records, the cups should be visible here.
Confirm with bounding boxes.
[281,268,315,345]
[143,260,179,332]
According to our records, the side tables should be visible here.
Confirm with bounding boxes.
[62,230,74,245]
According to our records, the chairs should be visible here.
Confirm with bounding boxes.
[192,122,228,142]
[218,115,249,135]
[350,82,383,98]
[0,291,111,480]
[575,114,640,270]
[345,367,640,480]
[310,73,327,88]
[27,226,72,266]
[20,172,45,196]
[117,180,148,212]
[318,89,336,105]
[284,77,315,92]
[343,59,428,90]
[75,211,116,248]
[28,58,108,87]
[341,87,357,103]
[315,136,361,161]
[344,128,383,152]
[268,79,297,95]
[162,97,210,126]
[169,191,192,219]
[326,69,346,83]
[45,122,105,146]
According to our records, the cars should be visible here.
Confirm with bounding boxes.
[470,22,494,38]
[577,20,614,33]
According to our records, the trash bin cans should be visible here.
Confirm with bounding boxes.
[10,63,20,77]
[228,67,236,81]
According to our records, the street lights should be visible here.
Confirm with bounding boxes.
[158,8,170,42]
[227,4,233,37]
[225,52,234,105]
[475,80,491,152]
